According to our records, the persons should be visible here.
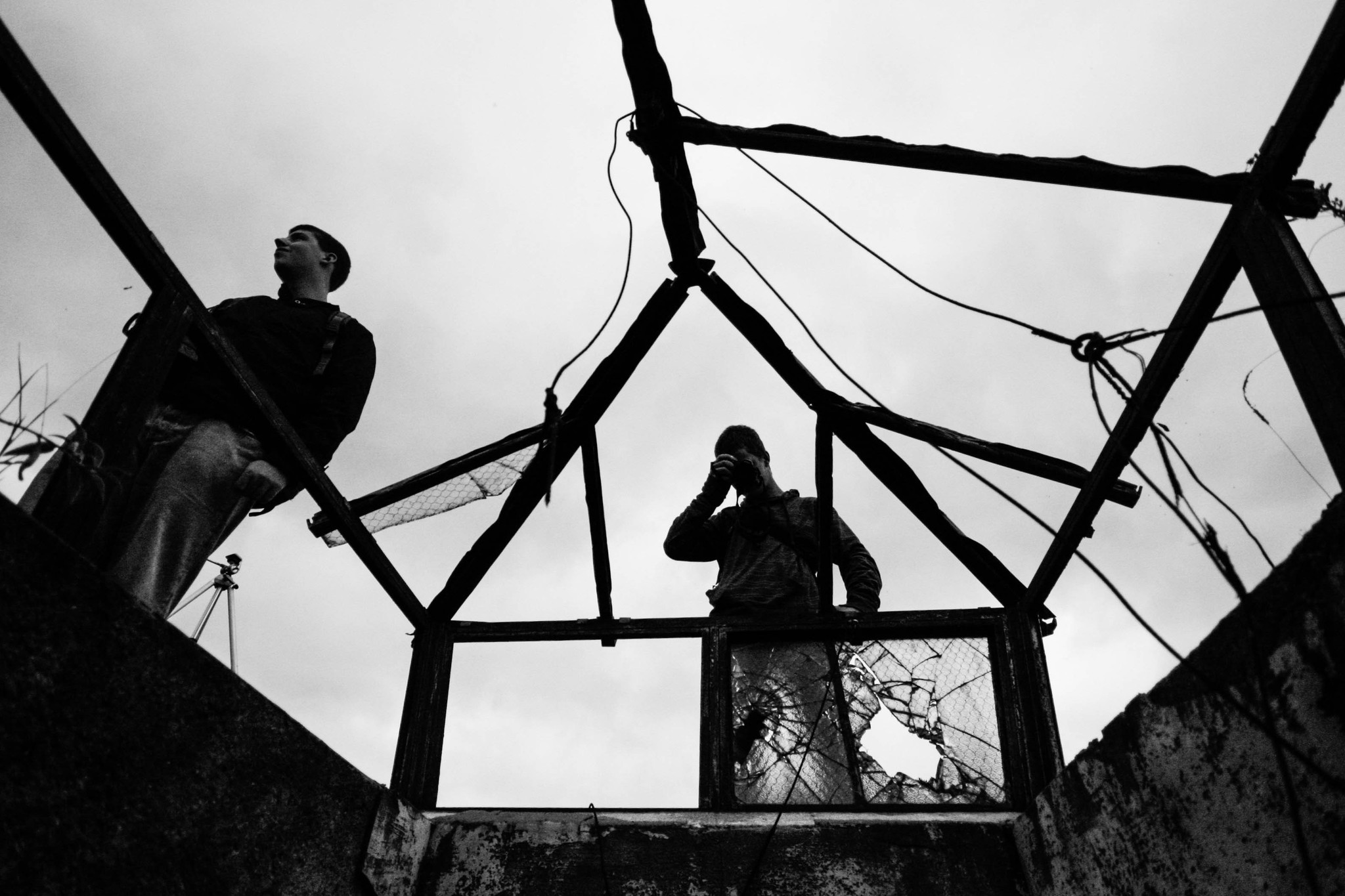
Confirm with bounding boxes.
[17,224,377,619]
[663,424,883,802]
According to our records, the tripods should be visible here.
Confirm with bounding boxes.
[167,554,242,675]
